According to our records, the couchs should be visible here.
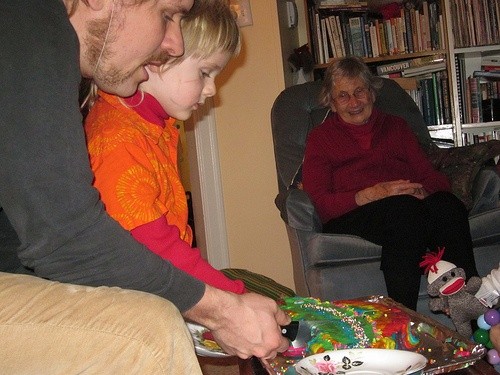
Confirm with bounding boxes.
[271,77,499,333]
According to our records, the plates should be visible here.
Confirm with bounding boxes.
[186,323,231,358]
[296,348,428,375]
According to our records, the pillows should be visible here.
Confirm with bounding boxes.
[432,140,500,213]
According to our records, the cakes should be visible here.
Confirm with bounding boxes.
[273,294,420,365]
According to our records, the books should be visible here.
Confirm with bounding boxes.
[309,0,445,64]
[452,0,500,47]
[377,56,500,164]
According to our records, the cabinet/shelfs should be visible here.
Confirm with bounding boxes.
[303,0,500,165]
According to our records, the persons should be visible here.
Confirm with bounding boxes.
[83,0,253,295]
[445,264,500,353]
[303,56,480,311]
[0,0,291,375]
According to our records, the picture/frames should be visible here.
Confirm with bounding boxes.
[229,0,253,28]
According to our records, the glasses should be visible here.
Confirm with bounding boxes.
[332,88,369,105]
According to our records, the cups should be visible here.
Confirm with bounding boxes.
[482,98,500,122]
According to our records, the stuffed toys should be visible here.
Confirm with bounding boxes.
[419,246,486,339]
